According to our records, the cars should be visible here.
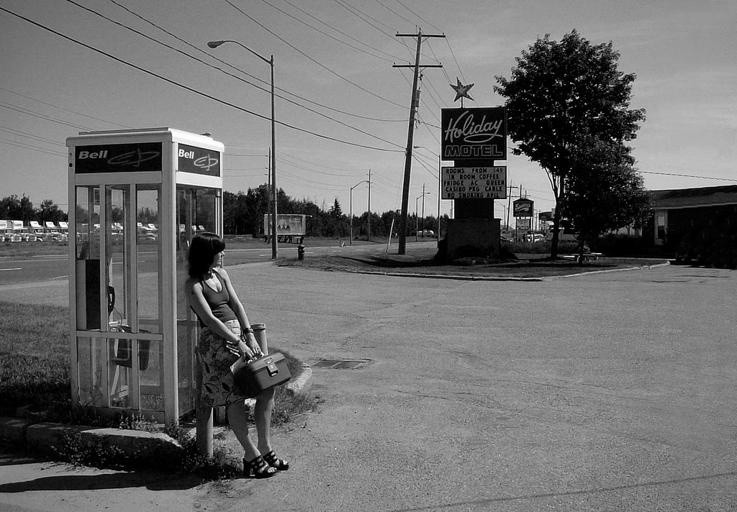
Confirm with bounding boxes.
[521,233,545,243]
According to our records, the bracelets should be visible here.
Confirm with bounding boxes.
[242,327,254,335]
[234,338,241,346]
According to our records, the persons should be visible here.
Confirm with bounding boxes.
[183,233,291,477]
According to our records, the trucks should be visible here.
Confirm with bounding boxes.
[0,218,207,243]
[546,226,579,242]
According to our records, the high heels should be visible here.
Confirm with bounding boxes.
[243,454,277,477]
[263,450,289,470]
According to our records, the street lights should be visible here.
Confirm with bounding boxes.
[349,181,372,246]
[207,40,277,259]
[415,192,430,241]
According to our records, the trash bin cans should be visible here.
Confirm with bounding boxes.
[298,245,304,260]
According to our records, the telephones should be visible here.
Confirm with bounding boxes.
[108,286,115,313]
